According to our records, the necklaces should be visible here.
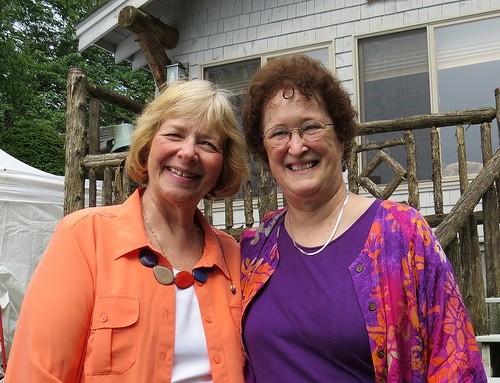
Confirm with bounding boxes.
[285,193,349,256]
[140,205,203,268]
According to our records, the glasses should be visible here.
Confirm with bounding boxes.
[261,119,334,147]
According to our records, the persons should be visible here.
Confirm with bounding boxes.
[1,79,249,383]
[236,51,490,382]
